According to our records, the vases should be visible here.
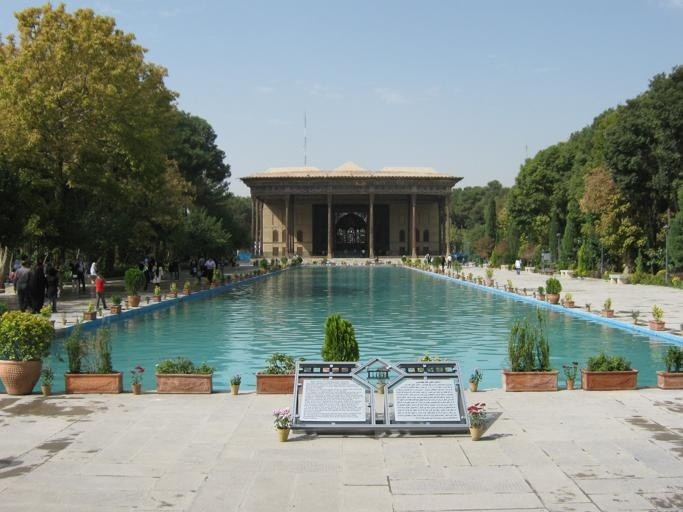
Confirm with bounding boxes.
[469,382,477,390]
[231,383,240,395]
[131,384,142,394]
[375,383,386,394]
[469,424,487,441]
[277,427,290,442]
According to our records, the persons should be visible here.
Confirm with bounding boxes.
[514,257,521,275]
[446,254,452,269]
[135,255,238,290]
[9,253,112,315]
[440,257,445,270]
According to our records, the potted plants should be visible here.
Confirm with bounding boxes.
[256,352,309,393]
[0,310,55,396]
[501,313,683,390]
[37,366,54,397]
[77,251,302,321]
[321,315,358,381]
[398,252,666,334]
[154,353,214,395]
[63,319,124,393]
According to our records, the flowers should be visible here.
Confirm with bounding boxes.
[129,366,146,385]
[466,367,482,382]
[270,408,298,429]
[462,403,487,429]
[227,371,244,385]
[375,366,389,384]
